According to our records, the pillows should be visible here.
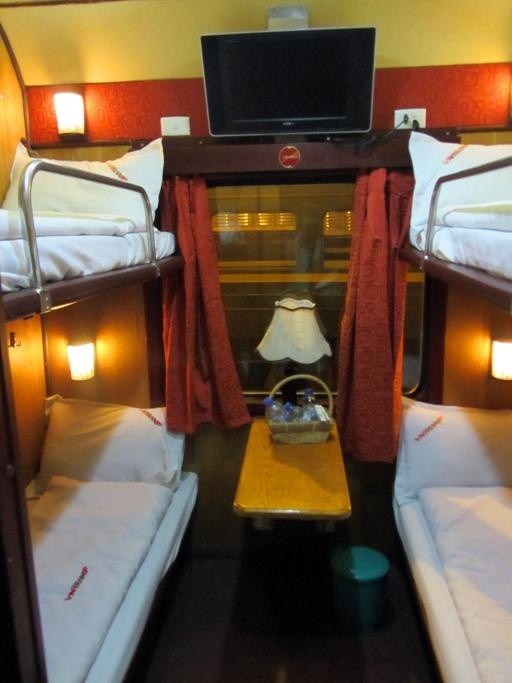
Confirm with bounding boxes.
[390,389,512,509]
[38,388,180,495]
[0,134,168,237]
[396,128,512,248]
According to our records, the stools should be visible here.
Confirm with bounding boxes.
[331,542,393,632]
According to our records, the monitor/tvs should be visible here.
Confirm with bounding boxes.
[200,26,376,137]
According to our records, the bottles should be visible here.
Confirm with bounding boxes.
[261,388,318,423]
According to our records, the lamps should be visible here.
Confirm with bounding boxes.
[491,333,512,385]
[255,295,335,404]
[51,86,87,141]
[66,335,97,384]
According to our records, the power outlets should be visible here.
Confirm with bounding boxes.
[393,108,426,128]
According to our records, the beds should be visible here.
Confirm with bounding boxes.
[0,450,199,683]
[0,198,180,328]
[398,430,511,683]
[395,217,512,304]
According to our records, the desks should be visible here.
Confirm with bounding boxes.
[233,417,353,537]
